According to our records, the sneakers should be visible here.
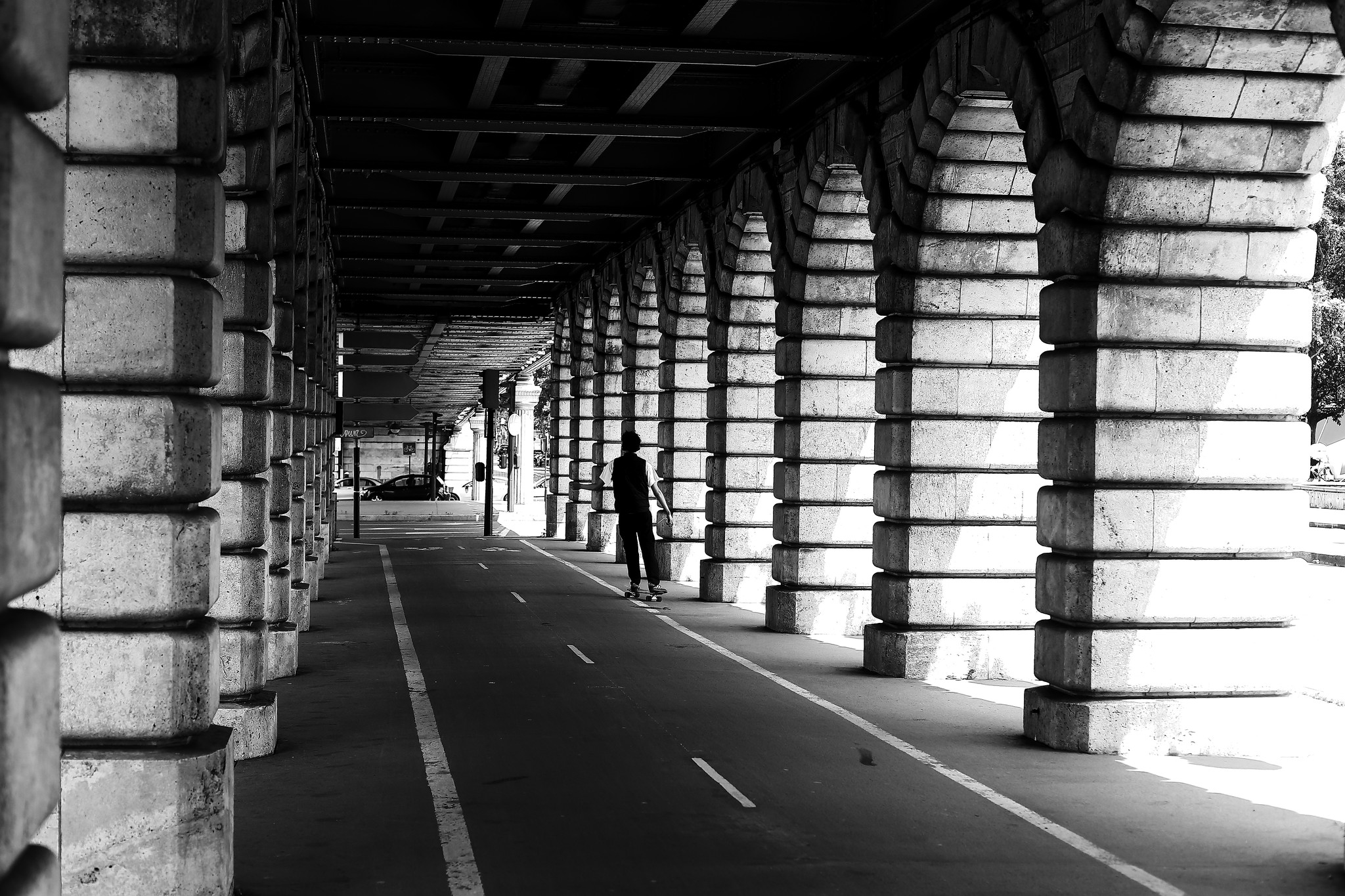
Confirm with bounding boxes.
[648,582,668,594]
[631,584,640,590]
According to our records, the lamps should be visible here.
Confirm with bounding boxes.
[387,422,400,438]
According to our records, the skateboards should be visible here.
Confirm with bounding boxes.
[624,587,668,603]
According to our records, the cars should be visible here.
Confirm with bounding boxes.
[359,473,449,502]
[332,476,381,500]
[494,444,552,467]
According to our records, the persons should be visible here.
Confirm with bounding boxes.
[568,430,674,594]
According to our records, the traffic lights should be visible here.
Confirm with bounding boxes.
[499,380,516,413]
[497,453,507,469]
[432,412,443,434]
[424,421,433,441]
[478,368,500,409]
